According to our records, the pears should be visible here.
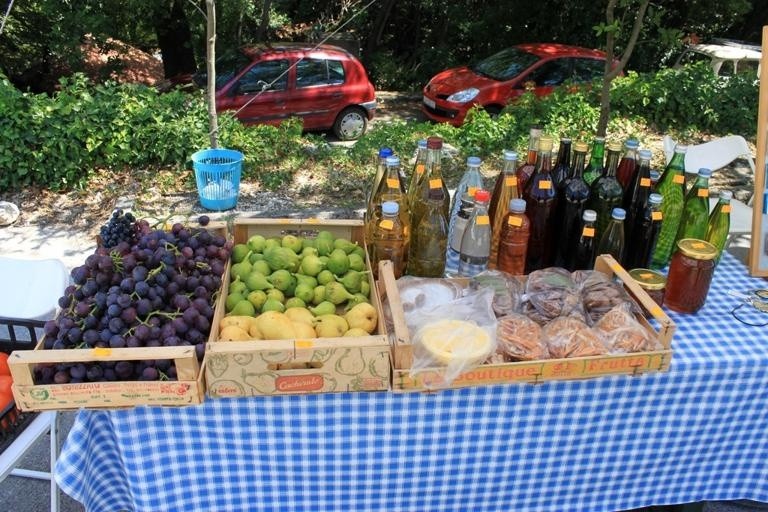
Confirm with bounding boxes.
[219,301,379,371]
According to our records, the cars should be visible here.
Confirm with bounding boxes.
[422,42,625,132]
[673,45,763,85]
[163,45,376,142]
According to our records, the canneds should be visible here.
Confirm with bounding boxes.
[623,267,667,322]
[664,237,720,315]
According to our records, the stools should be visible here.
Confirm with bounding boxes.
[663,135,758,204]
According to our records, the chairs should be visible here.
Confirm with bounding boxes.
[1,318,60,511]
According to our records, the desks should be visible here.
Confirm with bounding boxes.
[53,238,767,511]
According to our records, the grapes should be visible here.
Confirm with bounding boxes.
[33,209,235,385]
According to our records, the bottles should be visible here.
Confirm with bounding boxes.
[364,136,733,315]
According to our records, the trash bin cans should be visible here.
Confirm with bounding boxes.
[191,149,244,211]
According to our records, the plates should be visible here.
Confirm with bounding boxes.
[380,279,464,331]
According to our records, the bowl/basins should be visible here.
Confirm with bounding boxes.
[420,319,490,368]
